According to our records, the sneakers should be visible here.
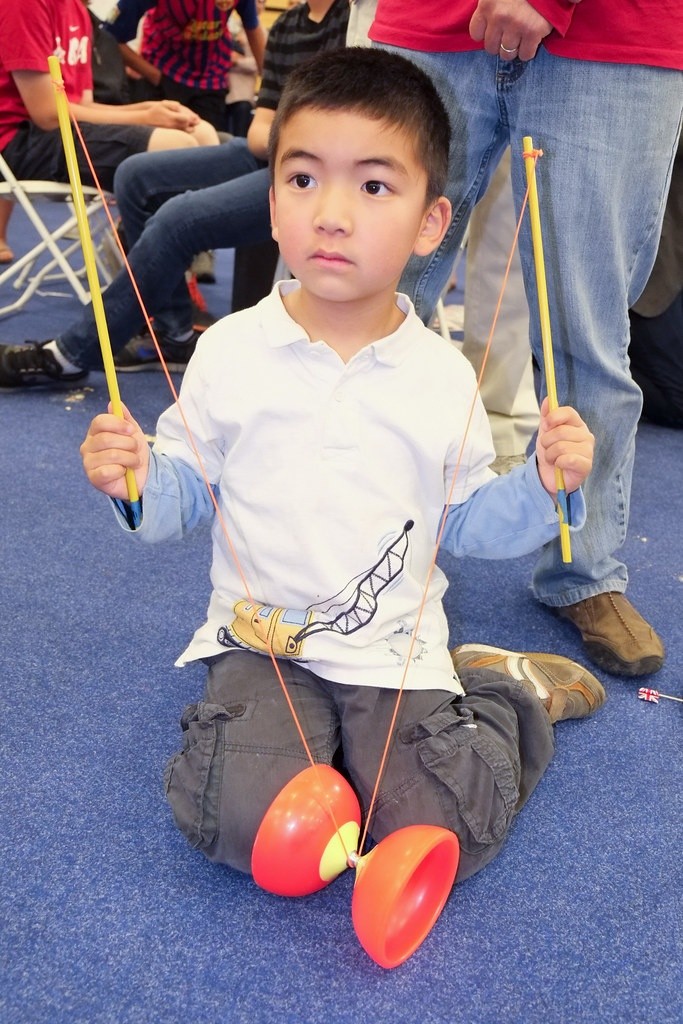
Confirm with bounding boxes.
[108,324,198,374]
[0,339,87,389]
[450,645,604,724]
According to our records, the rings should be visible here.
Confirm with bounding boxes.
[501,44,516,52]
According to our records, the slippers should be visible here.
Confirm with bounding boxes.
[0,239,14,263]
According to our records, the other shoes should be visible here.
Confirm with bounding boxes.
[191,250,215,283]
[553,592,663,676]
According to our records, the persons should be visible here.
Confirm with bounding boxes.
[346,0,682,677]
[1,1,351,389]
[80,47,606,884]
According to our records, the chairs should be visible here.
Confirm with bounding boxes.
[0,148,125,317]
